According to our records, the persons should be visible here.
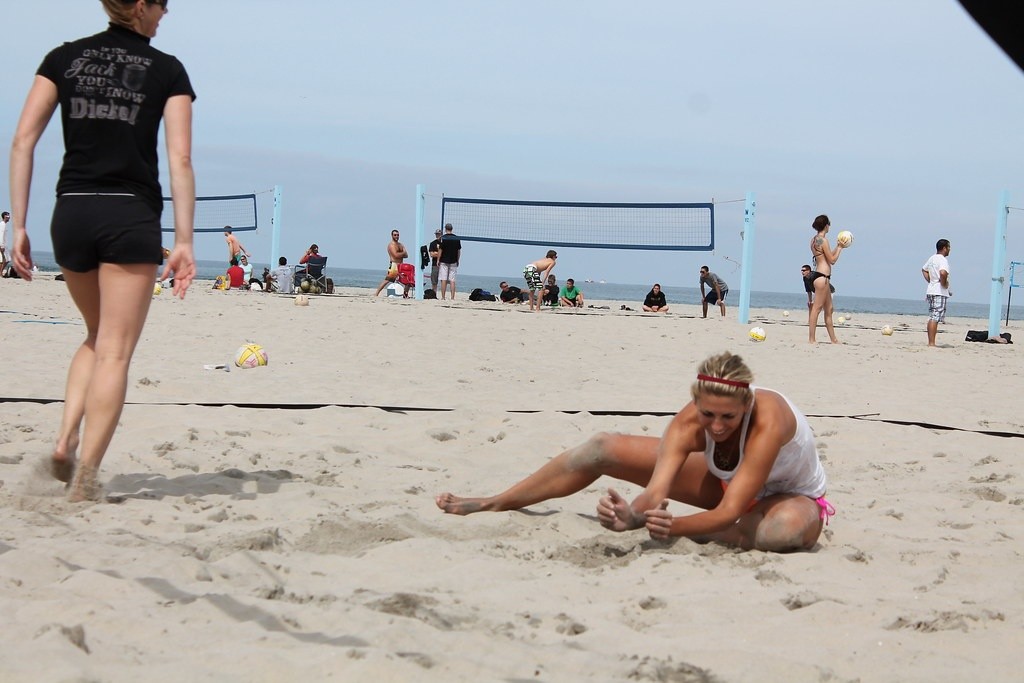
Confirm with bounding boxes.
[9,0,197,500]
[699,266,729,318]
[808,215,849,345]
[429,229,446,294]
[922,239,951,347]
[523,250,557,311]
[265,256,293,294]
[0,212,12,277]
[294,244,322,287]
[541,275,560,306]
[643,283,669,313]
[500,281,537,305]
[560,278,584,307]
[224,225,253,290]
[375,230,408,298]
[436,223,462,299]
[801,265,835,325]
[438,352,836,553]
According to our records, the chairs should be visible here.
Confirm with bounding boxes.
[289,256,328,295]
[394,263,426,299]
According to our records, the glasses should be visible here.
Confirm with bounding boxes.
[827,222,830,227]
[801,269,808,272]
[699,271,706,274]
[556,256,558,259]
[6,216,10,218]
[151,1,170,10]
[503,285,508,288]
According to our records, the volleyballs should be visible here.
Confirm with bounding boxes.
[881,325,893,336]
[294,295,309,306]
[165,248,171,258]
[235,343,268,369]
[837,317,846,325]
[838,230,854,248]
[749,327,766,342]
[298,280,320,293]
[783,311,789,316]
[153,282,161,295]
[845,315,851,320]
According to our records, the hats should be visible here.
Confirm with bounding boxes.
[310,243,319,254]
[434,228,443,234]
